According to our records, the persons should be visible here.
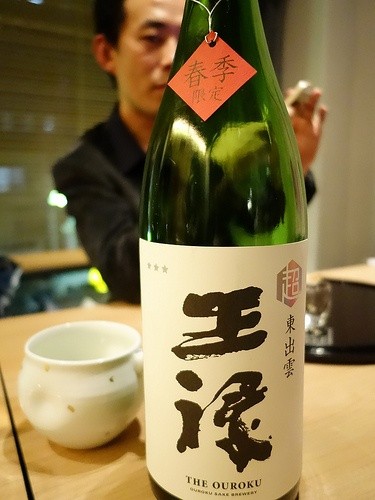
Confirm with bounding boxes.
[52,0,328,306]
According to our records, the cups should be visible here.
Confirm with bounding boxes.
[19,320,146,450]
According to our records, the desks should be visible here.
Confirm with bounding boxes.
[0,248,99,310]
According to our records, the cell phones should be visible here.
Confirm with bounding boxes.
[286,80,313,108]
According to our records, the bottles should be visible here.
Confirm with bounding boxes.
[131,0,311,500]
